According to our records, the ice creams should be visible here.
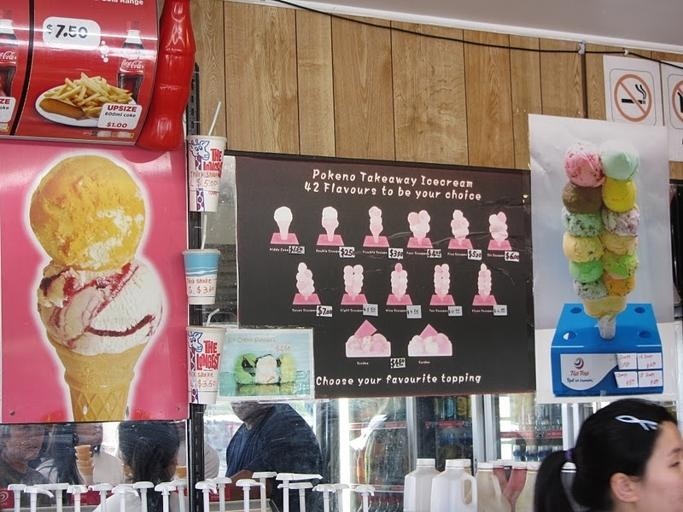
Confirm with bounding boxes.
[25,155,169,423]
[275,205,508,357]
[562,145,640,342]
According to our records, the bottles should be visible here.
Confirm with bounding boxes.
[118,19,145,104]
[0,8,17,98]
[0,18,17,98]
[118,29,144,104]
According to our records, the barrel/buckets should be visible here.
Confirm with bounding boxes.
[404,458,588,512]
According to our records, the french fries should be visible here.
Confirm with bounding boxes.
[44,73,132,118]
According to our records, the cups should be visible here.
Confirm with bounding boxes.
[181,249,220,306]
[185,324,227,406]
[186,134,227,213]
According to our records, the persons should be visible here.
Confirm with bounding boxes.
[225,402,326,512]
[0,422,219,512]
[534,399,682,512]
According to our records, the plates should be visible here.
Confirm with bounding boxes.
[35,83,137,128]
